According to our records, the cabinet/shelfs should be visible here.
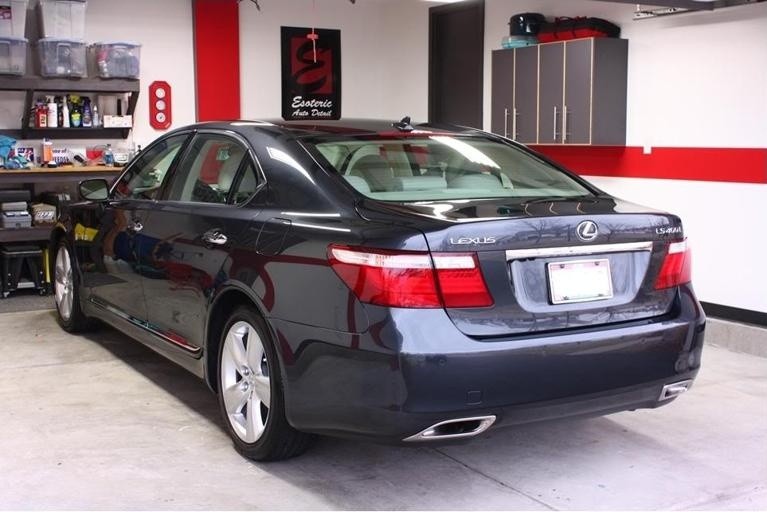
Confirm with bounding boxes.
[487,37,628,148]
[0,78,143,300]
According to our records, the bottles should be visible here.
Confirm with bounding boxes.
[92,103,99,128]
[104,144,114,167]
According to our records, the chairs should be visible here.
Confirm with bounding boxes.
[220,145,498,197]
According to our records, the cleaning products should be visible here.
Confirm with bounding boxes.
[80,95,93,128]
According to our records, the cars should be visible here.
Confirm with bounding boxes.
[49,117,707,462]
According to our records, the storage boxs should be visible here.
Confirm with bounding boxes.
[0,0,142,79]
